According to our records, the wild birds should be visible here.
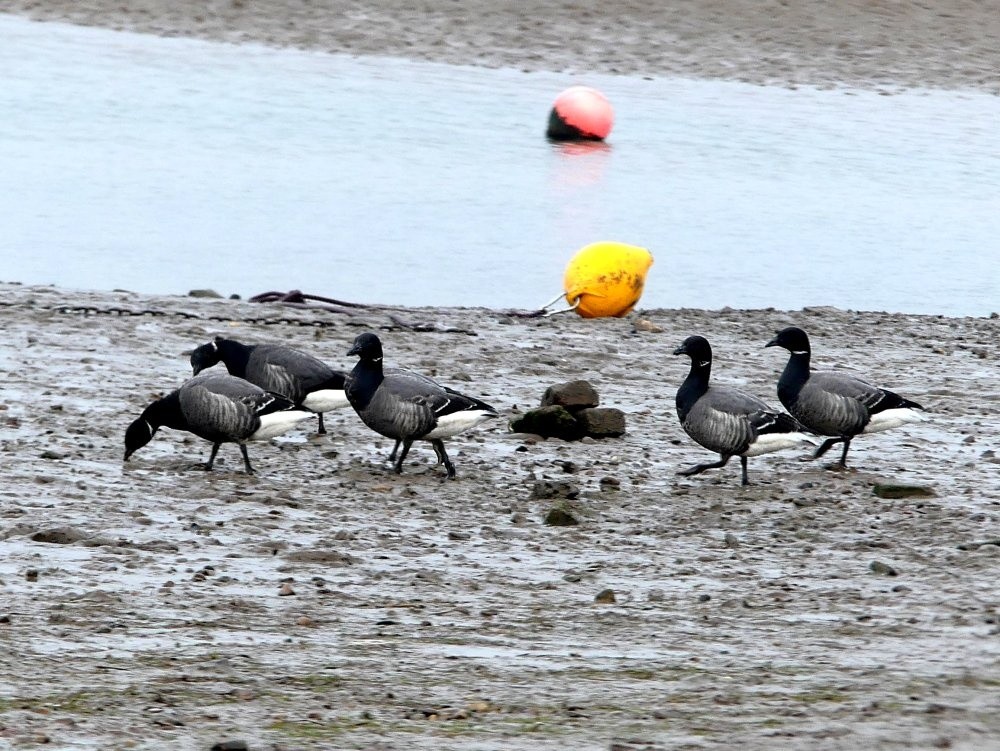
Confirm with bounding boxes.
[765,327,925,468]
[190,336,351,434]
[124,374,319,474]
[344,333,498,476]
[672,335,823,488]
